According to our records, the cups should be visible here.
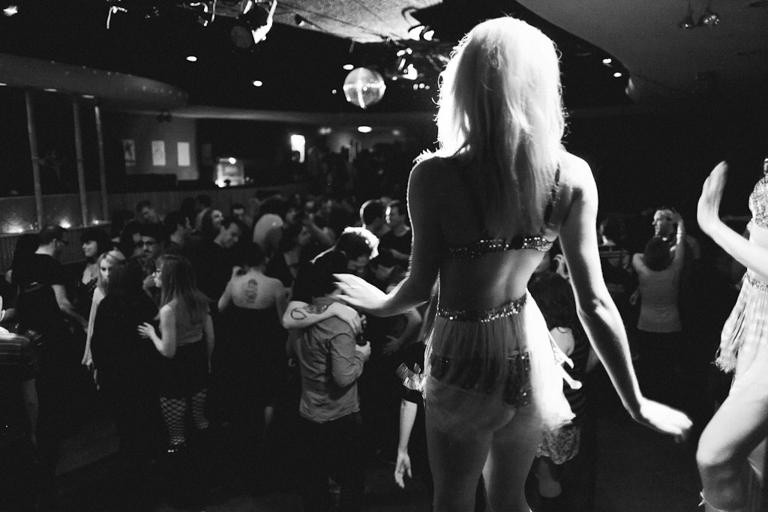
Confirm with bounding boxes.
[354,325,367,348]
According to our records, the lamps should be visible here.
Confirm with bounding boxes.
[342,67,386,109]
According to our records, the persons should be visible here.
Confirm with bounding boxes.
[382,283,422,356]
[377,200,413,269]
[527,248,593,376]
[81,229,115,306]
[138,253,215,456]
[251,186,360,242]
[341,200,386,259]
[696,155,766,512]
[230,203,245,225]
[632,209,686,369]
[217,242,289,430]
[0,295,30,343]
[393,279,440,488]
[164,211,192,256]
[266,222,311,302]
[333,17,693,511]
[202,208,224,242]
[652,208,677,247]
[599,216,633,334]
[140,225,171,302]
[199,216,246,300]
[5,232,39,285]
[298,253,372,498]
[137,200,159,224]
[18,224,89,480]
[81,251,147,480]
[336,233,373,275]
